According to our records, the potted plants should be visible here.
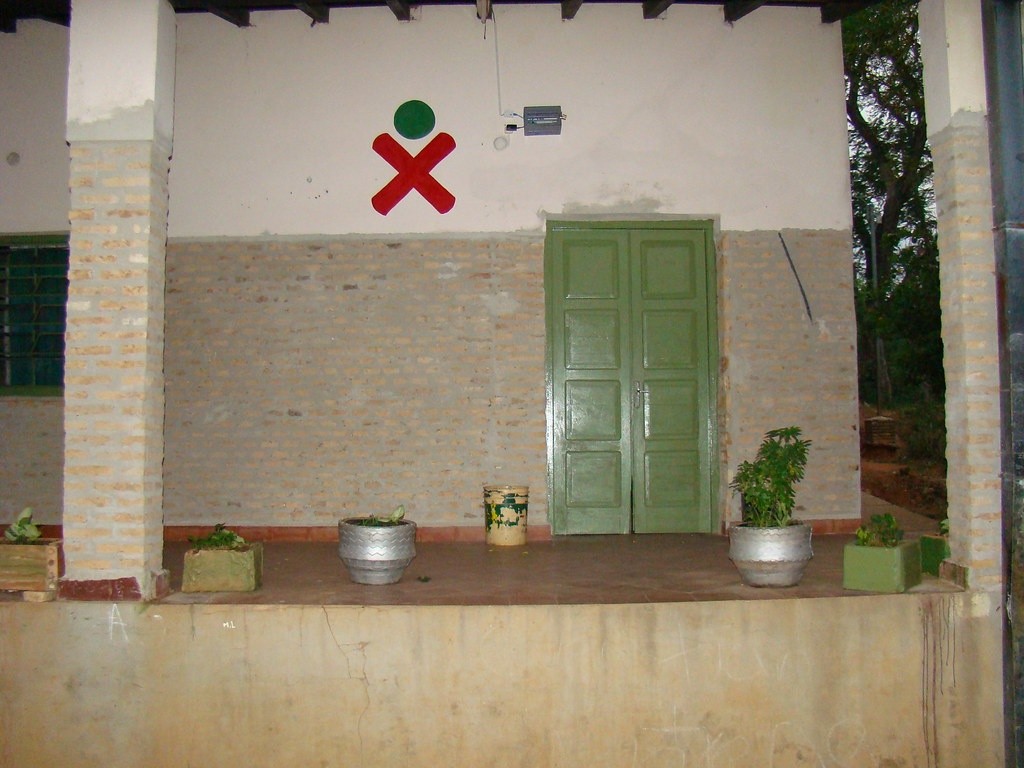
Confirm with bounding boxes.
[727,425,815,588]
[181,522,264,593]
[336,503,417,585]
[0,505,65,590]
[843,512,922,594]
[919,518,951,578]
